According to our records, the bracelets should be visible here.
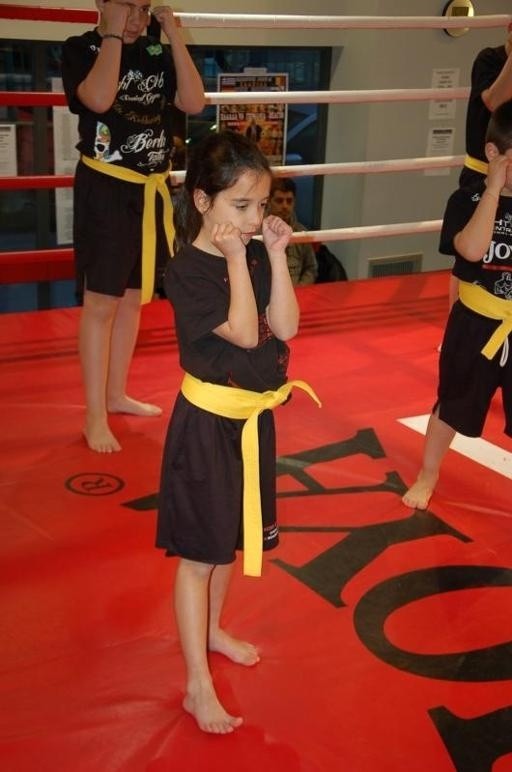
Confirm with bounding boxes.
[101,34,124,41]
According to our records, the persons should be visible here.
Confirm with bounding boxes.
[255,174,320,286]
[448,23,512,350]
[156,131,300,735]
[400,96,512,510]
[61,0,206,453]
